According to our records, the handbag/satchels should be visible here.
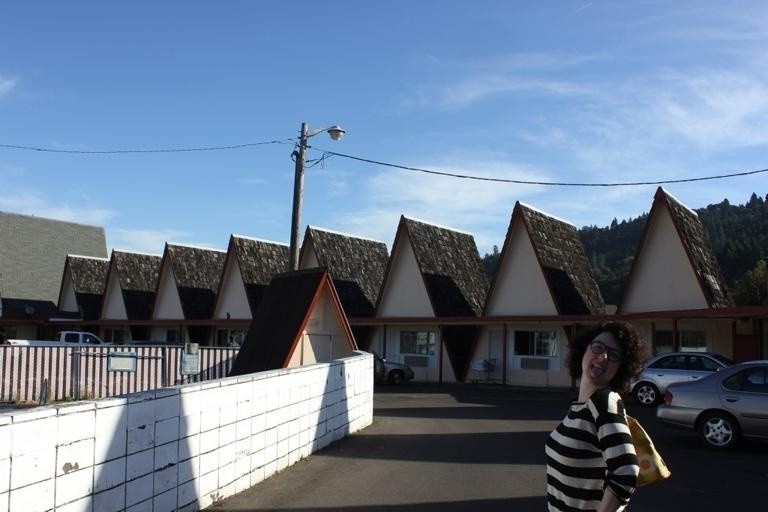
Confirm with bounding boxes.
[625,413,671,489]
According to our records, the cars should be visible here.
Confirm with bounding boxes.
[656,359,767,448]
[372,350,413,384]
[619,350,732,410]
[126,339,185,346]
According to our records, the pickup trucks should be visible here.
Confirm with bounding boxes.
[5,331,117,346]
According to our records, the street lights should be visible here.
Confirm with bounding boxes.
[289,121,347,271]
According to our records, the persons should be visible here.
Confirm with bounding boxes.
[544,319,652,512]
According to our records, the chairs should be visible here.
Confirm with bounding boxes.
[470,358,497,386]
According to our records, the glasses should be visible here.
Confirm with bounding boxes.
[589,340,622,363]
